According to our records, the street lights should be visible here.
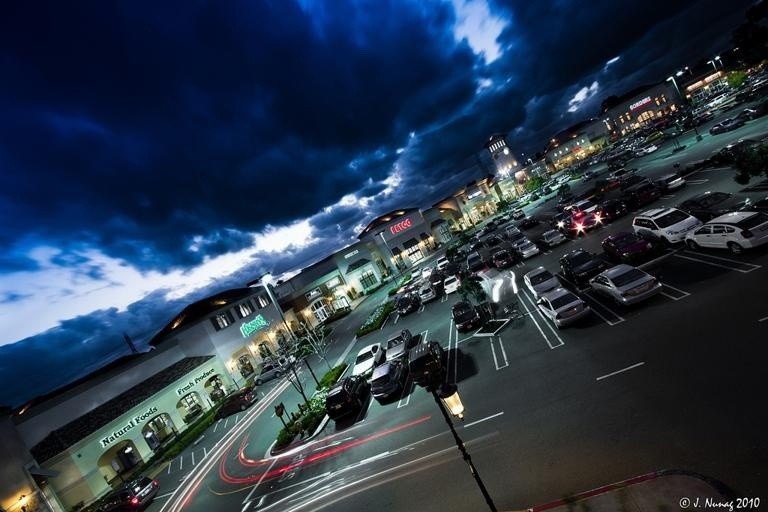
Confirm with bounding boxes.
[428,377,497,512]
[259,271,323,390]
[684,56,727,84]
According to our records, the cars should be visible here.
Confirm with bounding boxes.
[253,353,296,386]
[97,476,160,512]
[325,328,445,424]
[217,387,258,419]
